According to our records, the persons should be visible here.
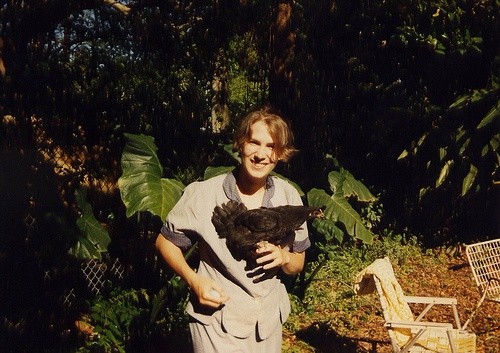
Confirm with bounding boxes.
[155,102,312,352]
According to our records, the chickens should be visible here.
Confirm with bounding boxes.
[211,201,325,261]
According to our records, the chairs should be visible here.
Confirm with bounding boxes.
[354,257,477,353]
[460,238,500,333]
